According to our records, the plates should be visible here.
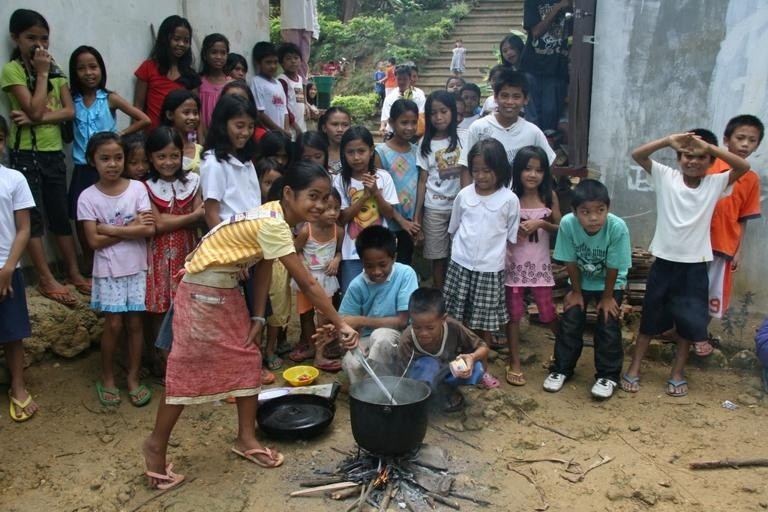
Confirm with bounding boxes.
[256,388,289,403]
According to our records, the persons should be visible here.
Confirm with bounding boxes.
[693,114,764,357]
[394,287,487,411]
[519,1,574,139]
[620,130,749,397]
[1,118,39,421]
[1,1,562,406]
[143,158,359,490]
[541,179,632,398]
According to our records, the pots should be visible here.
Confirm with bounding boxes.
[348,377,430,456]
[255,393,334,434]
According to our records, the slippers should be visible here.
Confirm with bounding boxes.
[693,339,714,358]
[7,387,35,421]
[231,442,286,468]
[665,367,688,397]
[620,370,641,392]
[96,378,122,406]
[262,347,343,385]
[75,278,93,295]
[151,350,166,383]
[129,380,151,407]
[442,363,527,412]
[37,280,78,306]
[143,461,185,490]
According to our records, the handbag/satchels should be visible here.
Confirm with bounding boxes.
[10,123,43,190]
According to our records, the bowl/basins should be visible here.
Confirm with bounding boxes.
[450,358,467,379]
[283,365,320,386]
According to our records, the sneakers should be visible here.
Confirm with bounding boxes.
[590,377,618,399]
[542,370,566,392]
